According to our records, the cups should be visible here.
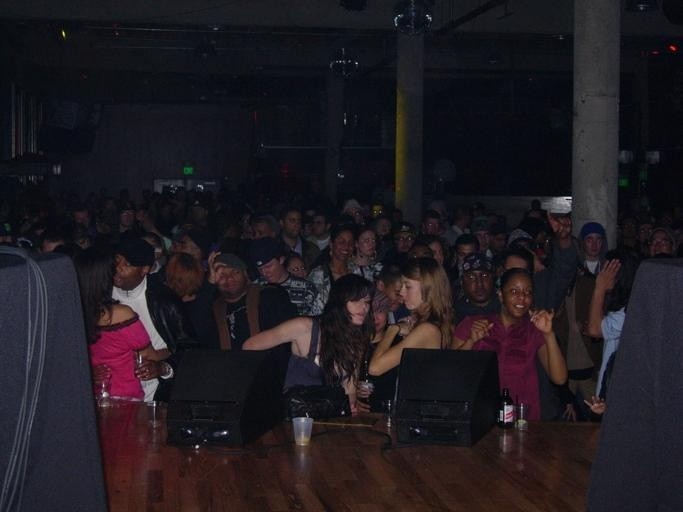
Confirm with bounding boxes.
[133,348,150,368]
[147,400,166,427]
[513,405,529,429]
[382,400,396,427]
[95,373,111,406]
[293,417,313,445]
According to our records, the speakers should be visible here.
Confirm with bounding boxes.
[165,347,285,448]
[393,348,498,446]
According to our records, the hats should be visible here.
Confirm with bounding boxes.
[392,221,415,232]
[507,228,532,246]
[249,237,278,266]
[581,222,604,237]
[648,225,676,246]
[371,289,391,312]
[118,238,154,266]
[463,253,495,272]
[213,253,247,270]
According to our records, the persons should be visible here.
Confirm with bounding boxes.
[113,238,188,402]
[368,200,386,219]
[649,226,675,258]
[548,233,608,421]
[576,222,610,321]
[446,235,481,280]
[392,221,416,252]
[418,235,444,264]
[284,252,307,278]
[637,223,654,243]
[586,347,617,413]
[358,289,402,411]
[440,204,471,242]
[243,273,372,414]
[453,268,567,422]
[369,256,453,377]
[73,245,170,402]
[522,199,547,237]
[497,245,533,273]
[258,208,322,268]
[307,225,372,317]
[305,213,333,248]
[341,198,365,223]
[470,216,497,249]
[452,253,500,323]
[584,258,625,399]
[347,225,381,281]
[373,216,392,239]
[167,253,217,346]
[247,236,319,320]
[421,211,442,234]
[211,254,296,382]
[0,186,253,254]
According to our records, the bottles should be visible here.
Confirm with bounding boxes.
[497,387,515,429]
[357,361,374,414]
[381,398,396,453]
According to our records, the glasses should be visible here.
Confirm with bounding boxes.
[648,238,672,245]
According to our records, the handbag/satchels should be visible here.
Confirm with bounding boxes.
[284,381,351,421]
